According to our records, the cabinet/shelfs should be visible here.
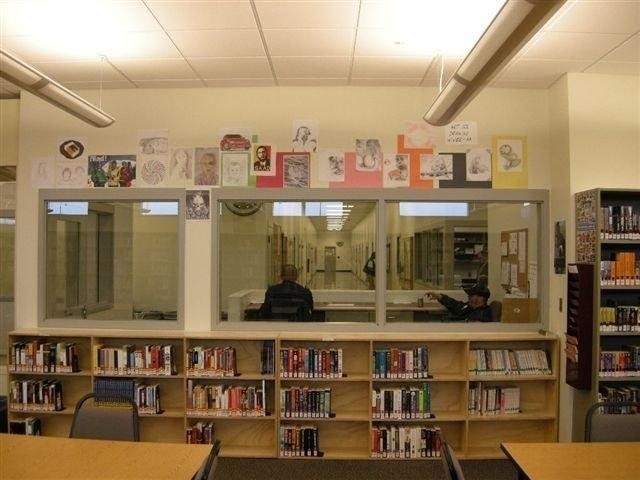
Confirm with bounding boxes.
[226,288,467,322]
[277,329,560,459]
[570,187,640,444]
[566,264,593,392]
[6,328,277,459]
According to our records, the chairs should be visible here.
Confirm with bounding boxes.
[69,393,140,442]
[194,439,223,479]
[584,400,640,443]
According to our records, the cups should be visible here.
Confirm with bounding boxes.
[418,297,423,307]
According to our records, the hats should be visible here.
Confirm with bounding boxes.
[464,285,490,298]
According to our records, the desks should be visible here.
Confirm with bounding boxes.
[489,300,502,323]
[499,441,640,480]
[266,292,308,322]
[1,433,214,479]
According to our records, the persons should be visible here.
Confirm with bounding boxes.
[424,285,492,322]
[226,161,242,185]
[387,155,408,182]
[363,252,375,290]
[197,154,217,185]
[91,160,136,187]
[172,149,189,183]
[254,146,270,171]
[256,264,313,322]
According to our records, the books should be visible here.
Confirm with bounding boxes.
[371,346,441,458]
[186,342,274,444]
[280,346,342,457]
[468,346,550,415]
[92,343,176,415]
[600,203,640,414]
[9,339,78,436]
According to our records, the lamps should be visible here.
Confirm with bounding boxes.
[423,1,568,126]
[0,45,117,128]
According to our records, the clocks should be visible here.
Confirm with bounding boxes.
[225,199,261,216]
